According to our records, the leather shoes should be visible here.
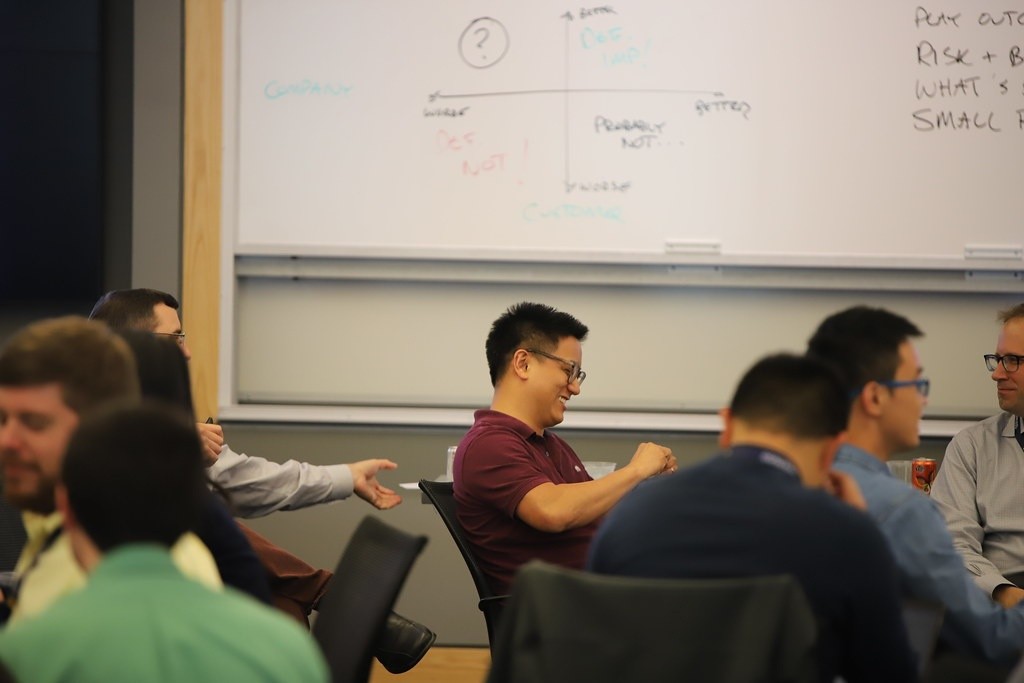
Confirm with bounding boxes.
[373,610,438,675]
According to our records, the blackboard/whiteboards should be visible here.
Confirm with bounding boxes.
[218,0,1024,269]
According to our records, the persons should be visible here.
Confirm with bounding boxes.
[454,299,679,639]
[927,303,1024,615]
[589,351,919,683]
[86,286,437,675]
[1,395,332,680]
[0,315,227,683]
[803,302,1024,681]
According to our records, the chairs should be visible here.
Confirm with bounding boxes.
[418,479,583,667]
[481,556,822,681]
[303,512,433,683]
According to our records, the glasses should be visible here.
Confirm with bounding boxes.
[984,354,1024,372]
[525,348,586,386]
[152,331,186,346]
[848,377,930,402]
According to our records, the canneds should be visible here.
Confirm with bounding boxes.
[913,458,936,493]
